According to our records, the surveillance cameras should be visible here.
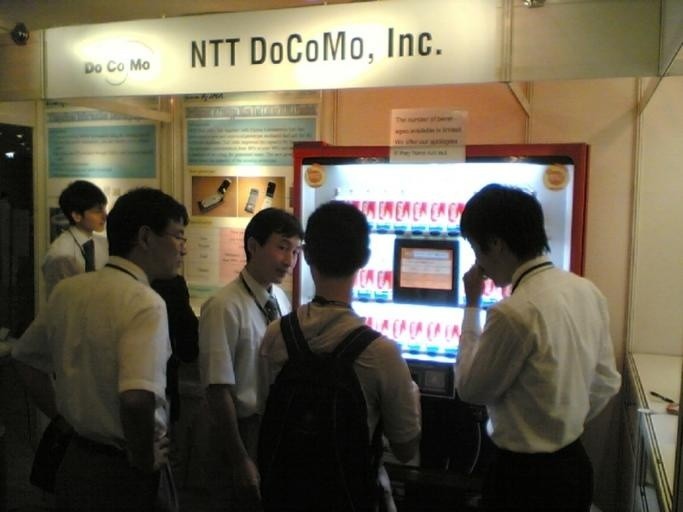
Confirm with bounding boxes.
[11,22,31,45]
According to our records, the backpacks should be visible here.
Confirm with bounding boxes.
[258,313,383,512]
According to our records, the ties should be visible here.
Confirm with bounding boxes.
[84,239,95,272]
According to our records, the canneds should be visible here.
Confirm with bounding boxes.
[341,200,466,224]
[357,269,393,292]
[481,277,497,299]
[365,316,462,349]
[502,283,512,297]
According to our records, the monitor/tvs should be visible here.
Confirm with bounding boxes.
[291,139,590,394]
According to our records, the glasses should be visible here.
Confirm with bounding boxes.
[161,230,188,248]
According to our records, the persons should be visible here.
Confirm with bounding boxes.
[41,180,108,303]
[12,186,190,512]
[253,201,422,509]
[148,272,199,461]
[198,207,302,512]
[455,182,622,512]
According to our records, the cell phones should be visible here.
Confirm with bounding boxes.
[246,188,258,213]
[261,181,277,211]
[199,178,232,208]
